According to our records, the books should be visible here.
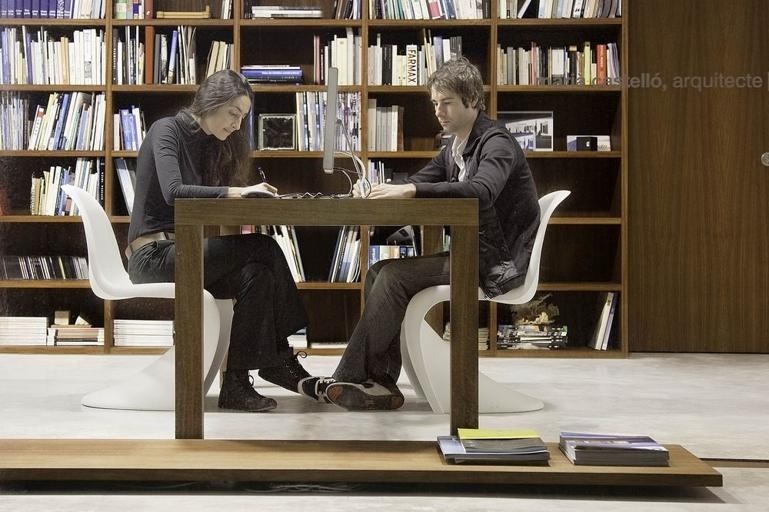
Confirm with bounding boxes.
[240,0,491,282]
[497,0,623,87]
[439,292,619,349]
[1,0,235,344]
[434,426,670,467]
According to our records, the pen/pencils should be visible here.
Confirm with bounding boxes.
[258,167,266,182]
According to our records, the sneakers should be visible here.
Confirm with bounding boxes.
[297,376,337,404]
[325,378,404,411]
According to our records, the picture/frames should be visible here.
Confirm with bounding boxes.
[494,108,555,153]
[257,111,297,151]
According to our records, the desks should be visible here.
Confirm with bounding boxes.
[172,195,482,441]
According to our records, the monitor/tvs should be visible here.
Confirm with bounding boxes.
[323,67,365,197]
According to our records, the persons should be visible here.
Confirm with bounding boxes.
[295,54,545,412]
[122,67,311,411]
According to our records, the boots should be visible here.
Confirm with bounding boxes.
[258,347,312,393]
[218,370,277,412]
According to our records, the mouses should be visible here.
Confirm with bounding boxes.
[241,190,274,198]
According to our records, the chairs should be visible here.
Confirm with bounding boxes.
[398,187,574,412]
[62,182,234,412]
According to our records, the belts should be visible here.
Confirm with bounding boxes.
[125,232,176,261]
